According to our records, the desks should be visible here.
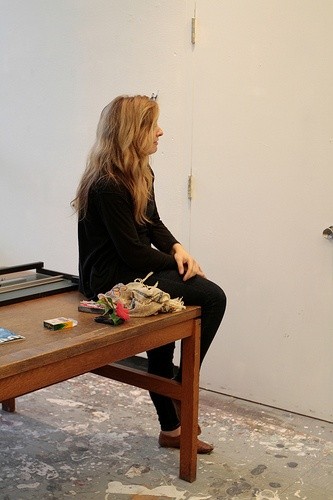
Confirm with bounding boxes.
[0,261,202,483]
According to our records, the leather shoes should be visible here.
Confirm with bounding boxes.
[172,377,201,435]
[158,431,213,454]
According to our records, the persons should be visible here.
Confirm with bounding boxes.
[70,95,227,454]
